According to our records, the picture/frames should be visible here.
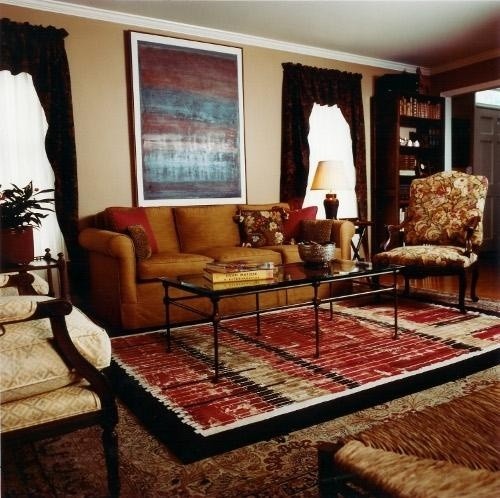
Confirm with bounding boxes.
[124,29,247,208]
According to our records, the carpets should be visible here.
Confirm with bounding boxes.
[103,286,500,465]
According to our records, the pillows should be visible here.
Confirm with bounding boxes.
[94,203,333,262]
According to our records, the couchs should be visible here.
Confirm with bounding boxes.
[78,212,356,332]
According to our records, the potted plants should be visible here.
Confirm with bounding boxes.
[0,180,55,263]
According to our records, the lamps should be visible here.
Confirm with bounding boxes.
[310,160,354,220]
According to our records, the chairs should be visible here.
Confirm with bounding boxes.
[0,273,121,498]
[0,271,50,296]
[373,170,488,314]
[317,381,500,498]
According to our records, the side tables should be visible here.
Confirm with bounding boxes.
[339,218,375,261]
[0,248,67,300]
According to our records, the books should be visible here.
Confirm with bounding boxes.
[399,155,416,232]
[203,259,273,284]
[202,278,276,290]
[400,97,440,120]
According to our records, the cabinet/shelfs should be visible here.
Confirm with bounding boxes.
[370,95,445,248]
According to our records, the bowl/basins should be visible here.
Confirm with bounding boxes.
[297,242,336,268]
